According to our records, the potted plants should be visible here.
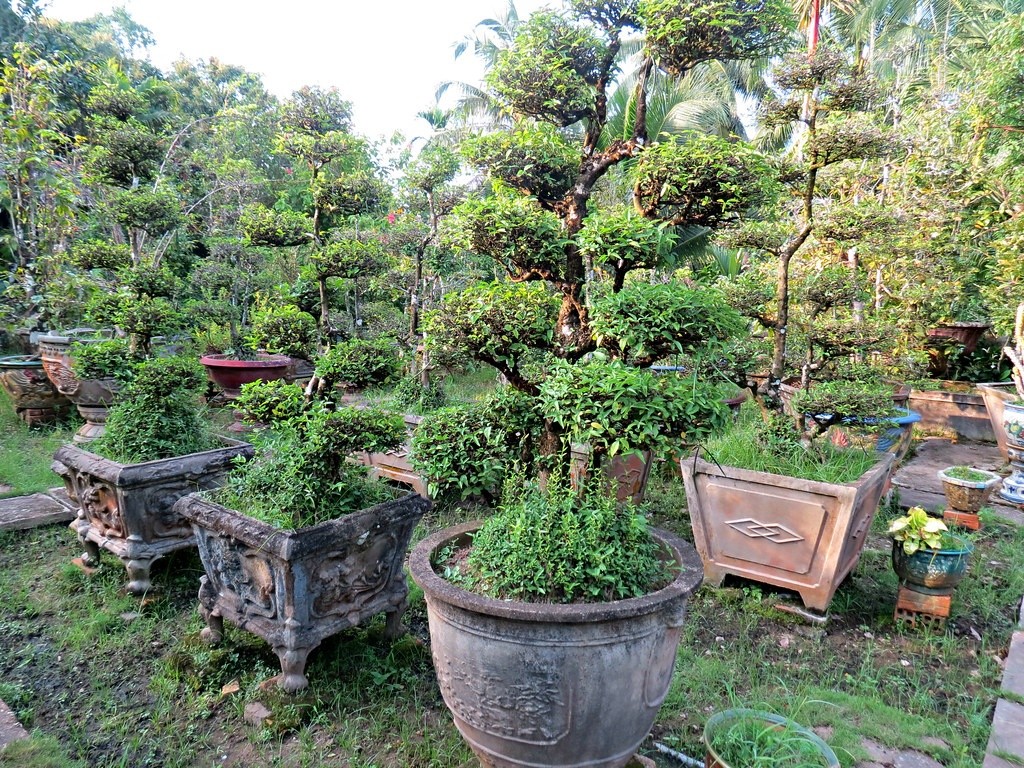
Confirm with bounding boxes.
[977,381,1024,459]
[889,506,972,596]
[715,382,748,424]
[38,285,171,407]
[539,437,657,515]
[49,72,253,596]
[173,84,422,693]
[778,373,911,414]
[679,45,911,616]
[911,301,992,376]
[938,466,1002,513]
[704,707,839,768]
[999,401,1024,504]
[333,403,454,500]
[189,159,295,434]
[409,0,791,768]
[805,406,921,474]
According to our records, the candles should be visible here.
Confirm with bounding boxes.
[0,44,71,408]
